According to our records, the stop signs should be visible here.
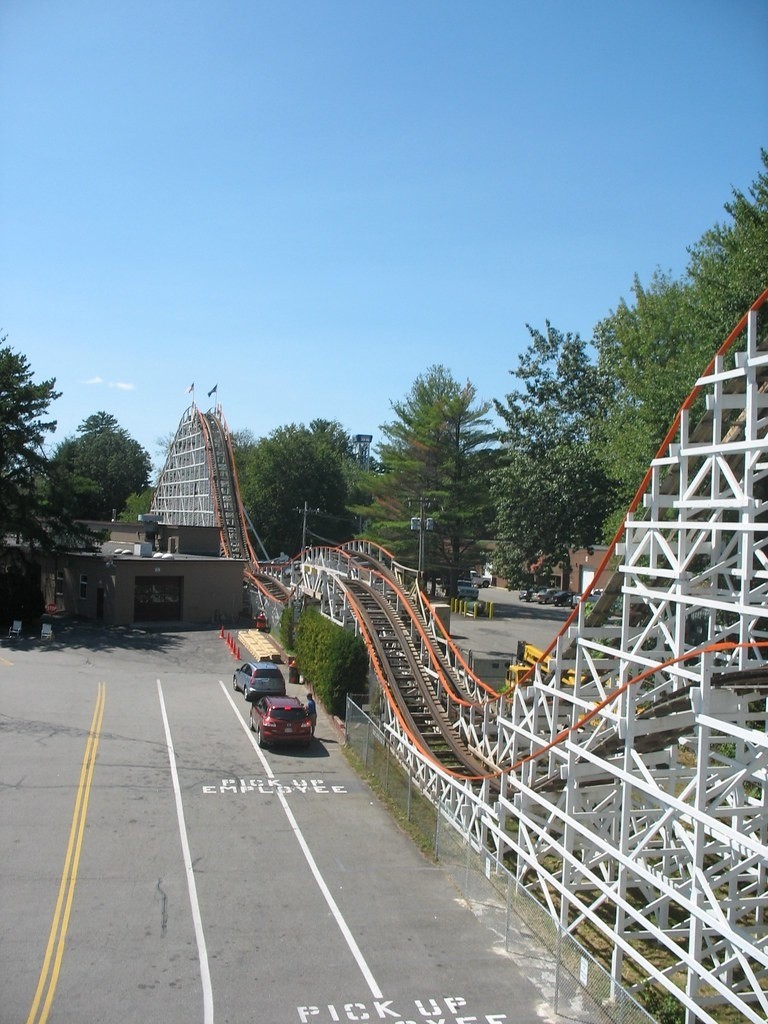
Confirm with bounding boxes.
[45,602,58,615]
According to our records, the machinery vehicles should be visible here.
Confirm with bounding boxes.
[504,640,647,738]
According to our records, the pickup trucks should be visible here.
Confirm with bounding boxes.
[572,588,606,605]
[518,587,548,601]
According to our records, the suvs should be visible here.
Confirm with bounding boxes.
[232,661,286,701]
[248,694,312,749]
[456,580,480,601]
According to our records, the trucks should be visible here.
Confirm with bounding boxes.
[436,569,491,588]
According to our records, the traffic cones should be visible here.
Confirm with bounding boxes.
[229,637,234,649]
[225,632,231,645]
[230,641,236,655]
[235,647,241,661]
[219,626,224,639]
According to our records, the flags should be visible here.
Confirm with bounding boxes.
[184,383,194,393]
[208,384,217,397]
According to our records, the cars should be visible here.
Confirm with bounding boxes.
[535,589,559,604]
[551,591,578,606]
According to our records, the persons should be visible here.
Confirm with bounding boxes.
[305,694,317,736]
